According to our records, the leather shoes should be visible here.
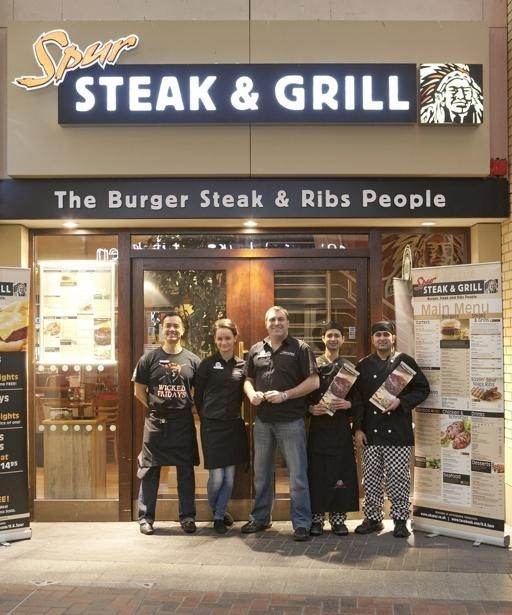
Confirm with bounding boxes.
[181,521,197,533]
[140,523,155,535]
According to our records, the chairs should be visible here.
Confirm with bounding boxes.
[106,413,119,465]
[46,376,67,396]
[97,404,118,417]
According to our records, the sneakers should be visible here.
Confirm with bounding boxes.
[393,519,411,538]
[310,523,324,535]
[241,521,273,533]
[354,519,384,534]
[332,525,349,537]
[213,520,227,534]
[224,511,234,526]
[292,528,309,541]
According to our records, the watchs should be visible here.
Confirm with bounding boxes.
[282,392,288,401]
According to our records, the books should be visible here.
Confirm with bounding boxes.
[318,363,360,416]
[369,360,416,412]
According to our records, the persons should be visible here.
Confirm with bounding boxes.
[306,321,356,535]
[423,72,481,124]
[130,313,201,533]
[194,318,249,535]
[243,305,321,542]
[353,321,430,538]
